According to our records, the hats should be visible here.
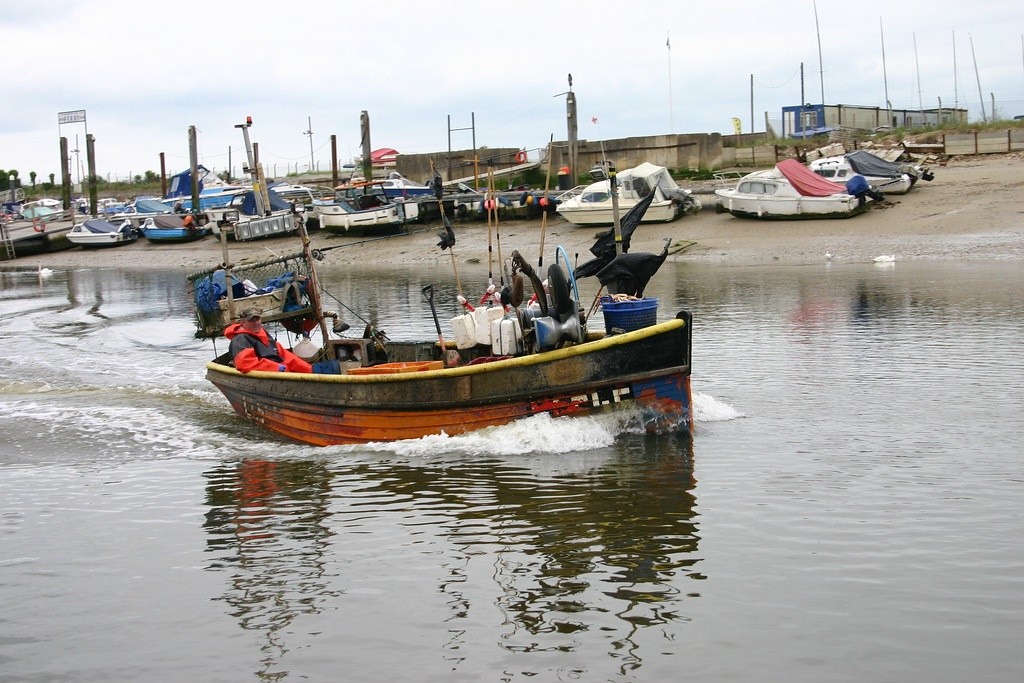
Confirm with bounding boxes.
[239,306,262,322]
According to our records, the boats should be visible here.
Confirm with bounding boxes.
[0,171,532,245]
[555,160,704,225]
[204,132,694,448]
[714,157,886,221]
[809,148,935,194]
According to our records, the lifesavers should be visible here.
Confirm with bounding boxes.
[33,218,46,232]
[515,151,527,163]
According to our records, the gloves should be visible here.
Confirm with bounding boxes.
[279,365,286,372]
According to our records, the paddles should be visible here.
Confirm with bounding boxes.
[422,284,465,369]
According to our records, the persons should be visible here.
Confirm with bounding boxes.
[184,211,198,233]
[225,306,312,374]
[290,198,306,223]
[801,148,808,164]
